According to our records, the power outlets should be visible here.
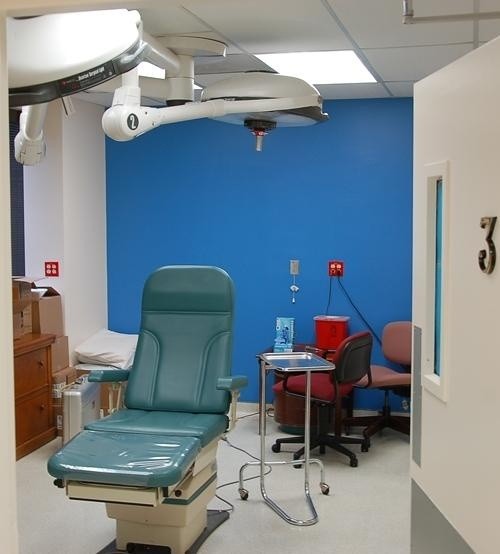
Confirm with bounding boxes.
[329,260,343,276]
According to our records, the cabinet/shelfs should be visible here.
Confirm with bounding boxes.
[14,333,56,461]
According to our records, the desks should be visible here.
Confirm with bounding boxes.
[255,343,336,435]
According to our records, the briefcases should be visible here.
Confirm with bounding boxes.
[63,374,101,444]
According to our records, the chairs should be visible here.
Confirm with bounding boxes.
[354,320,411,447]
[47,266,248,554]
[272,331,372,468]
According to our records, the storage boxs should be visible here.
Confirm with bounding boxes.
[32,282,78,437]
